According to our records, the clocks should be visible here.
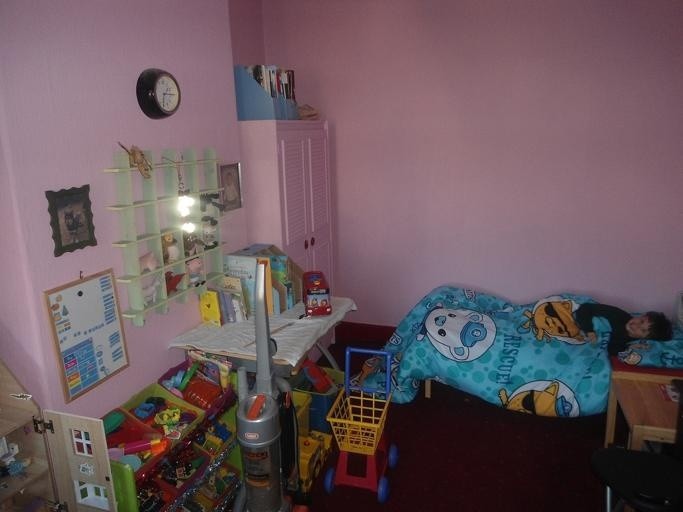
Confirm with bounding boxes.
[135,68,180,120]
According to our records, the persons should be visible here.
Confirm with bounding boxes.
[572,297,673,354]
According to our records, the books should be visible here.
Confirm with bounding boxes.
[244,62,296,102]
[197,239,305,327]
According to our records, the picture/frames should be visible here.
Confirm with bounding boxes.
[44,184,97,257]
[42,268,129,404]
[216,161,244,214]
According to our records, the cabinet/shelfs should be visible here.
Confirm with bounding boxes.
[102,147,227,328]
[239,118,335,296]
[1,363,242,511]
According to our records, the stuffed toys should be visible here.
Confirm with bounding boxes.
[116,138,153,179]
[139,188,227,306]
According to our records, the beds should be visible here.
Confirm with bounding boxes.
[425,288,683,399]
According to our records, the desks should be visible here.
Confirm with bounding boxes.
[604,371,682,451]
[173,296,353,377]
